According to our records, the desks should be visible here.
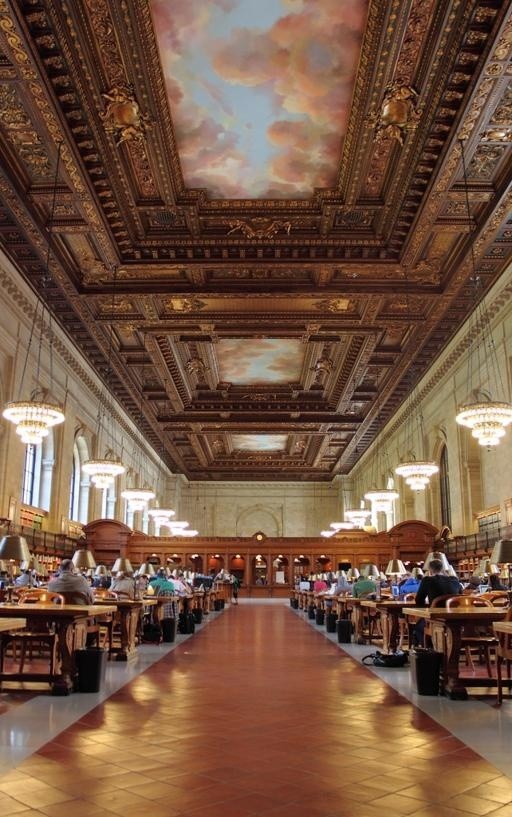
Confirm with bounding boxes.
[290,589,512,706]
[1,589,216,695]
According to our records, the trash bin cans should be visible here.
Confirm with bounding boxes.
[290,597,299,610]
[192,608,203,624]
[180,614,195,634]
[215,599,225,611]
[337,620,352,642]
[316,609,325,626]
[307,605,315,620]
[142,624,160,646]
[326,613,338,633]
[70,650,103,694]
[160,618,175,643]
[409,648,443,696]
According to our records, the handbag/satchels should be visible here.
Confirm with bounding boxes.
[362,649,408,667]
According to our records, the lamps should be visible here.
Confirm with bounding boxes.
[306,551,458,582]
[319,428,353,537]
[148,379,175,524]
[398,263,440,493]
[82,262,126,489]
[364,333,400,513]
[456,139,511,451]
[2,140,66,446]
[0,535,205,607]
[346,378,371,527]
[160,435,199,535]
[122,332,155,512]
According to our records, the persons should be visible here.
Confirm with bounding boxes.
[0,558,239,648]
[296,547,508,647]
